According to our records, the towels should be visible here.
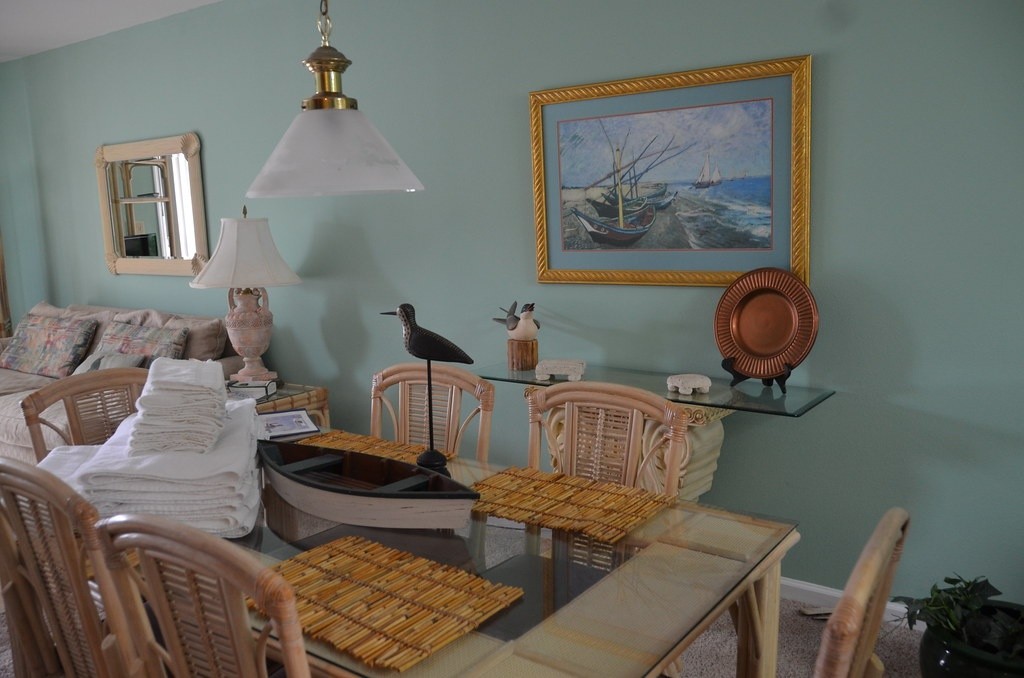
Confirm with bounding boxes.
[31,355,271,540]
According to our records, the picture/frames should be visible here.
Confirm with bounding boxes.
[530,54,812,290]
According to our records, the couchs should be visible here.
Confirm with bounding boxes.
[0,303,246,466]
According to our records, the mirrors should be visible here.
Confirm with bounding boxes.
[94,132,209,276]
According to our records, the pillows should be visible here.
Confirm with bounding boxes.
[95,321,192,369]
[0,312,98,379]
[30,301,115,363]
[71,351,144,375]
[112,309,228,361]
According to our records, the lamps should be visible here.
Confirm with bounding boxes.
[246,0,426,197]
[189,206,303,384]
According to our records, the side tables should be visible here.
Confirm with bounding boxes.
[256,383,332,429]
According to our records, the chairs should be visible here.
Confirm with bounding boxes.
[813,506,912,678]
[0,456,313,678]
[322,363,496,575]
[18,366,150,464]
[474,381,691,643]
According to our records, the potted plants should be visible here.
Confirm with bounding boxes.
[881,573,1024,678]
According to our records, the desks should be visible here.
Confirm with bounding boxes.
[479,359,836,502]
[88,429,802,678]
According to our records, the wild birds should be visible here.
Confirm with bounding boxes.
[493,300,540,341]
[379,303,474,364]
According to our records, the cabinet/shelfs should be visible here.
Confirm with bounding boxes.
[111,156,176,259]
